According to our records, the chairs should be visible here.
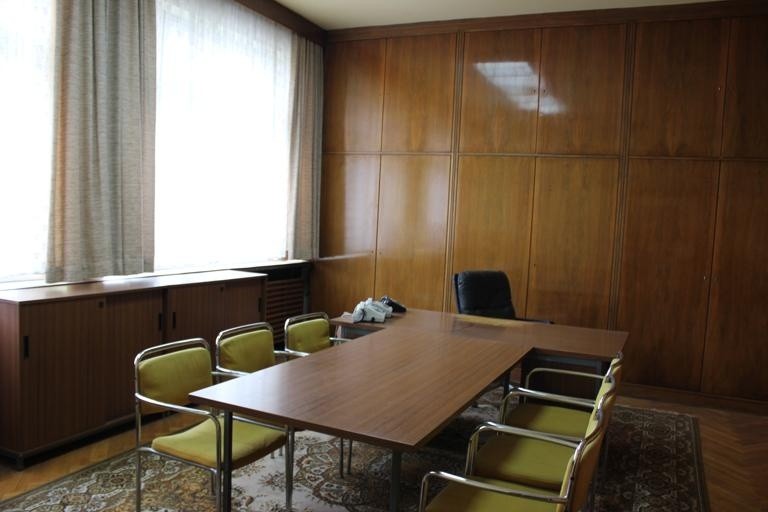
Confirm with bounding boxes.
[215,321,343,508]
[469,373,618,492]
[454,270,556,408]
[418,407,605,512]
[133,337,292,512]
[501,350,625,443]
[278,311,353,475]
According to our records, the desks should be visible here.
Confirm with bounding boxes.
[328,305,632,467]
[187,326,534,512]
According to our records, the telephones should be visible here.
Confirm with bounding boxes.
[352,298,393,323]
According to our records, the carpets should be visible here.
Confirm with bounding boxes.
[0,381,712,512]
